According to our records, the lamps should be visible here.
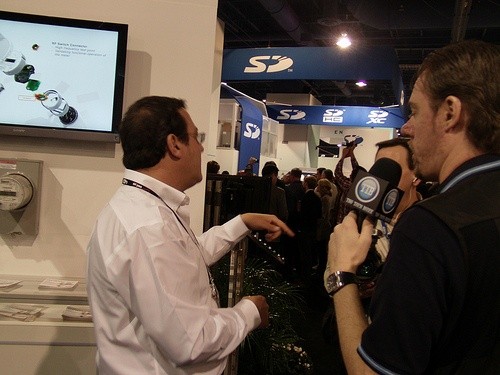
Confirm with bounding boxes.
[335,15,352,49]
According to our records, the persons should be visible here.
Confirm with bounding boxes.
[326,38,500,375]
[86,94,296,375]
[207,139,500,375]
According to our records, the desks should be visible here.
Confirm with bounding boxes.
[0,274,97,375]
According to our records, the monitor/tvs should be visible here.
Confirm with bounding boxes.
[0,10,128,143]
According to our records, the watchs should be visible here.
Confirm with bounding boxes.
[327,269,355,298]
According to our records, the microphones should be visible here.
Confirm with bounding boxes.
[346,137,363,147]
[345,157,402,233]
